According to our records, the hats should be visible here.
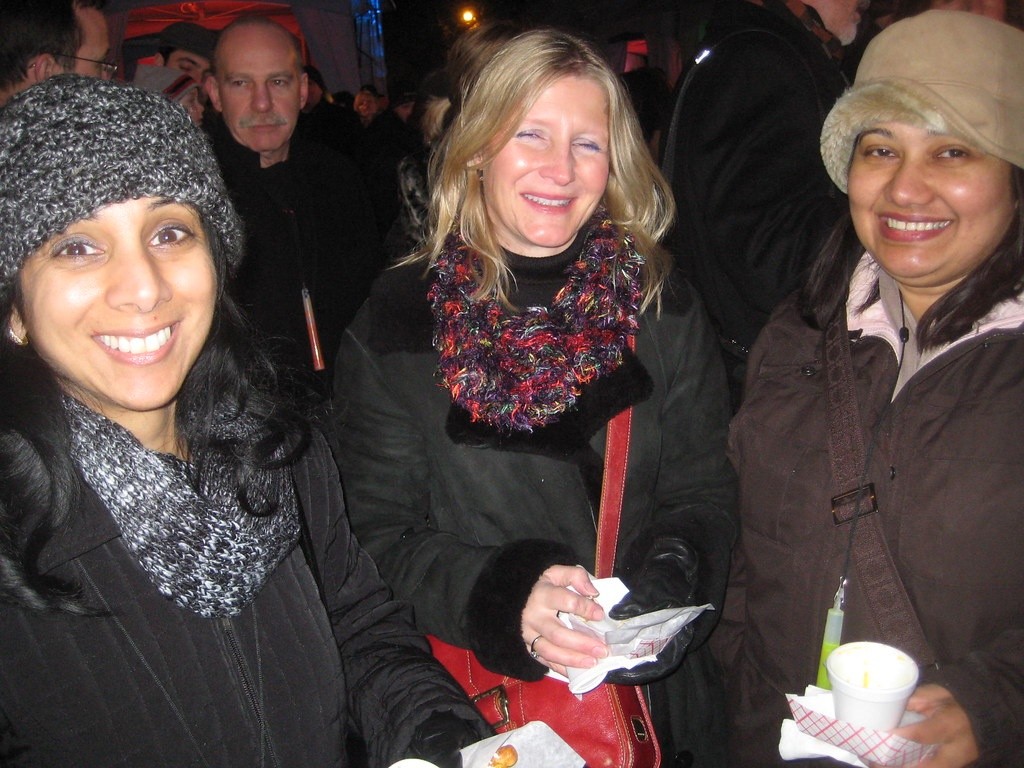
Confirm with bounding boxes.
[820,10,1024,195]
[132,66,202,104]
[0,73,243,305]
[157,22,218,61]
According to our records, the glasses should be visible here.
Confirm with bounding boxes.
[31,54,118,80]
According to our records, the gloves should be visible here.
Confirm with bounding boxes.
[605,533,702,685]
[411,712,481,768]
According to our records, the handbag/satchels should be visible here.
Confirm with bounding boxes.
[425,635,661,768]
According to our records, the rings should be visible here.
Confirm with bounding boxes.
[530,636,542,658]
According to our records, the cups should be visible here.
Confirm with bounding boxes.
[825,640,921,735]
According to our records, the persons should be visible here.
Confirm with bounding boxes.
[0,0,1024,768]
